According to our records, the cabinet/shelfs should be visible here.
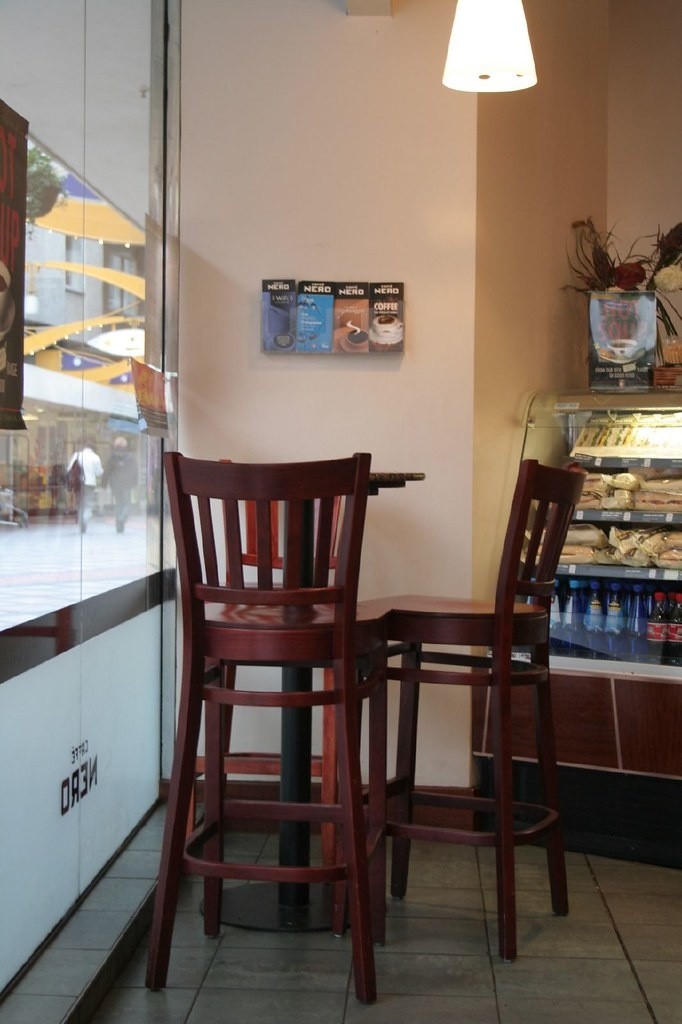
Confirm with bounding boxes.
[473,388,682,871]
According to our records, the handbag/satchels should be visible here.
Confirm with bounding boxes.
[66,452,84,492]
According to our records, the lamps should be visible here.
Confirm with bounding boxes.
[441,0,536,92]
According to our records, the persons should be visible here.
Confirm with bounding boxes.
[68,440,105,533]
[103,438,140,533]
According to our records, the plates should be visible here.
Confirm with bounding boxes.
[340,339,369,352]
[599,349,646,364]
[369,327,403,345]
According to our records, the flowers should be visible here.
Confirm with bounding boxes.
[559,214,682,365]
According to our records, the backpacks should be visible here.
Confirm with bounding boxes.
[110,453,130,487]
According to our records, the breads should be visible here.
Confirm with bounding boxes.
[526,471,682,570]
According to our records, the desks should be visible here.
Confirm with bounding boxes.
[198,472,425,934]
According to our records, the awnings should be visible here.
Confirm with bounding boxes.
[107,418,141,435]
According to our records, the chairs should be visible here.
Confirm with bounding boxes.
[361,461,589,962]
[184,455,336,877]
[143,451,379,1004]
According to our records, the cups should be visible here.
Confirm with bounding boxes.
[272,333,297,352]
[345,330,369,348]
[373,315,403,338]
[607,340,641,358]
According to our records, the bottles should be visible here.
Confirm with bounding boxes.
[662,329,682,367]
[525,577,682,666]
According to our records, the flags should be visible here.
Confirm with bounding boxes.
[130,357,169,438]
